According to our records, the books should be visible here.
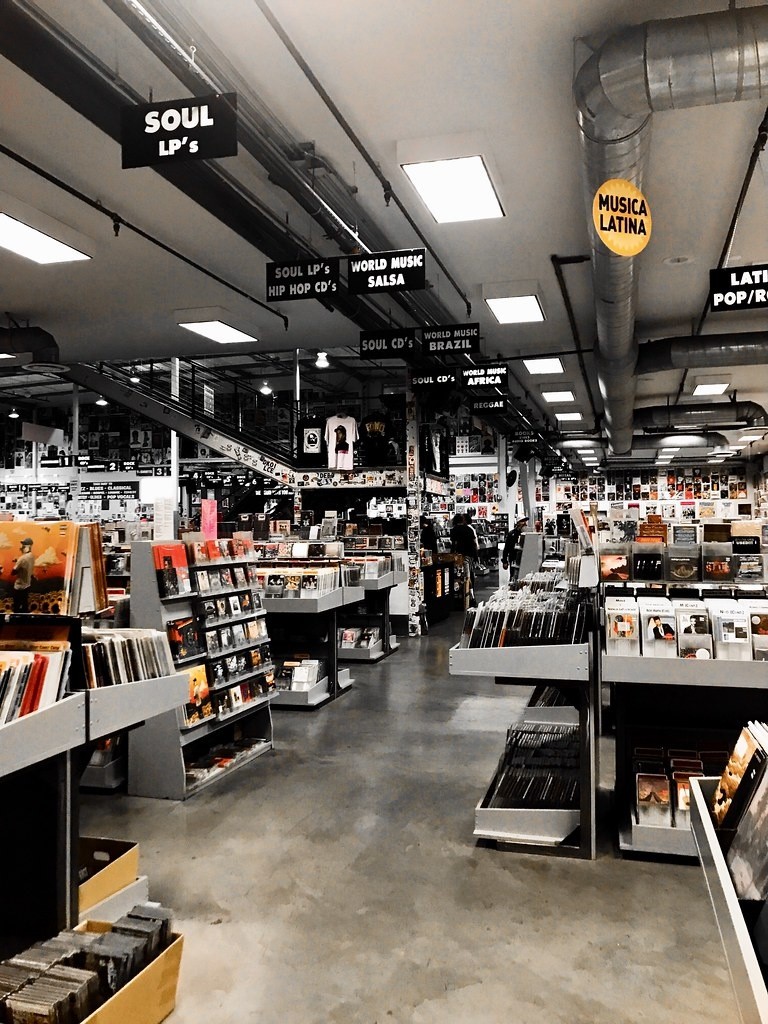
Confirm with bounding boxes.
[0,466,768,1024]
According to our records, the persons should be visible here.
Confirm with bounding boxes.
[572,528,578,539]
[420,522,438,553]
[501,515,529,582]
[683,615,707,634]
[462,514,480,550]
[450,514,478,607]
[652,616,675,639]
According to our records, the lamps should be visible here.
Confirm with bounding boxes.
[540,382,576,403]
[559,424,588,433]
[0,189,96,266]
[689,374,731,396]
[553,406,583,423]
[480,279,549,326]
[394,133,508,224]
[171,307,259,345]
[519,346,567,375]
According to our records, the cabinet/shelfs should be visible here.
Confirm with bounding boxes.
[0,470,768,1024]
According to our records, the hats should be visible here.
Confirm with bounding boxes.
[334,425,346,434]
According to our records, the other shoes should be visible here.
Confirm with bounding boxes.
[469,598,478,607]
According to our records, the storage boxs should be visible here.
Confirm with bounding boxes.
[59,919,183,1024]
